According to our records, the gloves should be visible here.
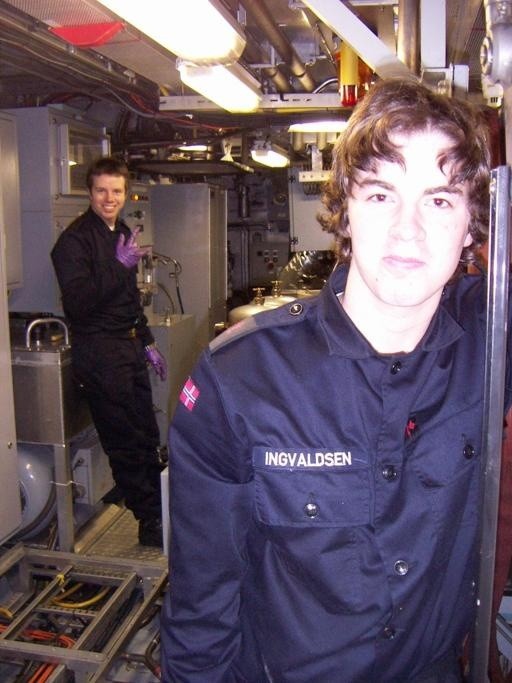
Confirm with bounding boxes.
[145,348,167,381]
[114,226,149,269]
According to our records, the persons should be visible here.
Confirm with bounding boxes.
[49,155,167,548]
[158,76,512,682]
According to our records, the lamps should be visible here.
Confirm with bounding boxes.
[100,0,247,66]
[175,54,262,113]
[249,138,290,169]
